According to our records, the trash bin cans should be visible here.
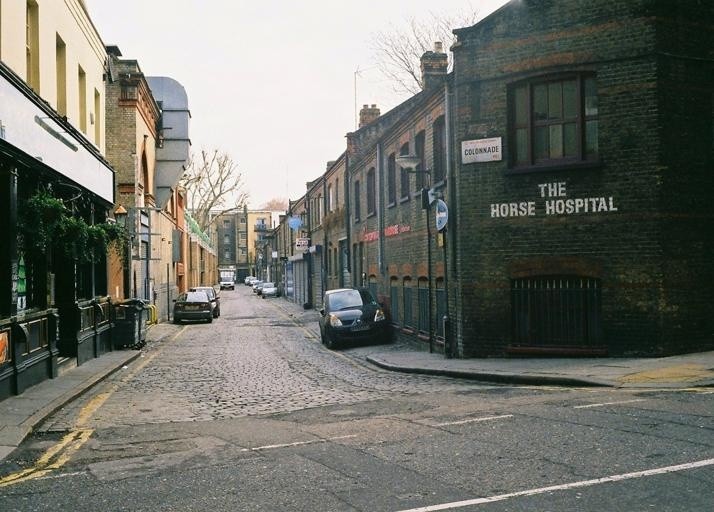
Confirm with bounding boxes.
[111,298,149,351]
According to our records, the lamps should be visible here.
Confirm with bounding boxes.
[394,154,432,177]
[114,204,129,229]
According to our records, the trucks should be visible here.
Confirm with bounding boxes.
[218,268,237,290]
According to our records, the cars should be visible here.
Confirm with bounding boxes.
[172,286,221,323]
[245,276,280,298]
[318,286,394,350]
